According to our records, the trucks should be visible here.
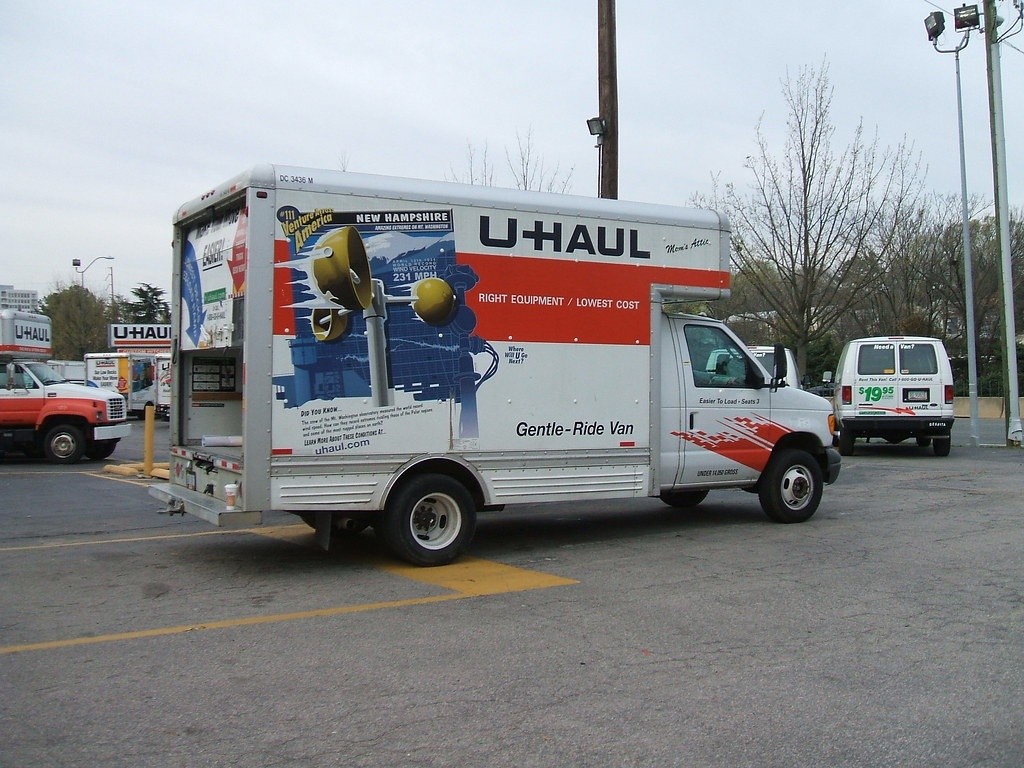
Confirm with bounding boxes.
[147,161,843,567]
[0,309,132,465]
[83,323,171,423]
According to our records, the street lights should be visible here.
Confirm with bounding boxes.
[924,4,984,449]
[72,255,115,360]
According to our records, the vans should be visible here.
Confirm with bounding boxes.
[706,344,811,390]
[823,336,956,456]
